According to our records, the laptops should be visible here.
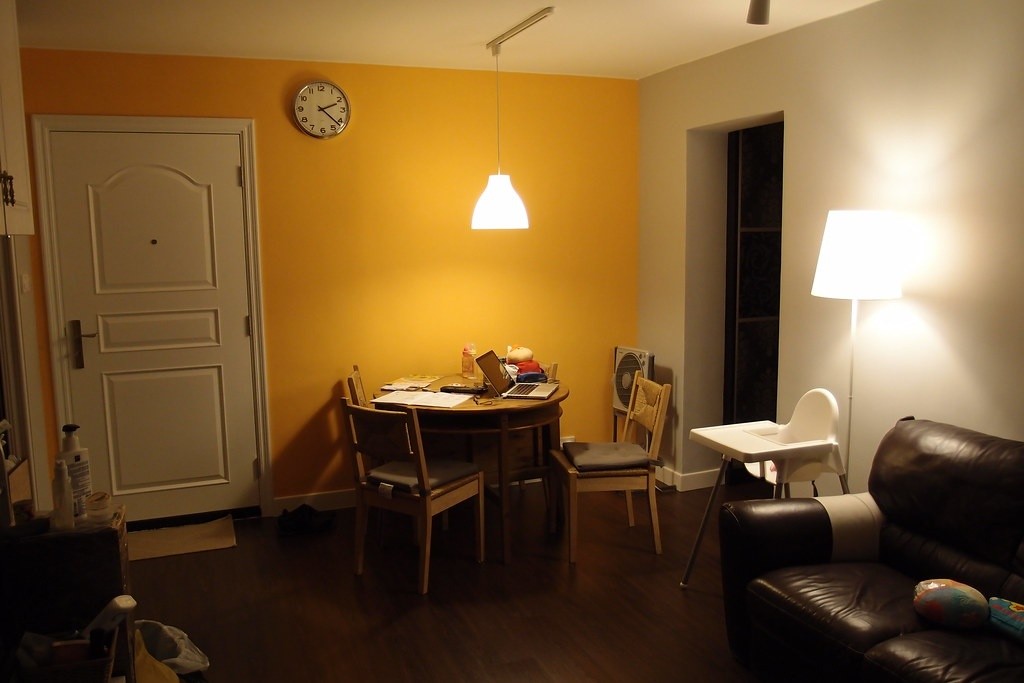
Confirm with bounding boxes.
[474,350,559,399]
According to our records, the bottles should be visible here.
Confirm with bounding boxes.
[79,594,137,640]
[52,459,75,529]
[467,342,478,380]
[461,346,468,377]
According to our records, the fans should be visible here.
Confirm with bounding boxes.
[612,346,677,497]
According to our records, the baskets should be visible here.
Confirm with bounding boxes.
[15,641,117,683]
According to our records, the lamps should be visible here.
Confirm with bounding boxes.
[811,208,904,480]
[471,45,529,230]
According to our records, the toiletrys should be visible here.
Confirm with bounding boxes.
[57,423,96,521]
[52,460,74,532]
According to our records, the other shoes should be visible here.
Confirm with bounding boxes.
[278,505,337,536]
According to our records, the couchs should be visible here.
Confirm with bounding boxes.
[713,417,1024,683]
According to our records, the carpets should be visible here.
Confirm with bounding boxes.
[123,514,238,561]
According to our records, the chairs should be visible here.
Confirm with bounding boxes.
[679,420,851,597]
[340,365,484,592]
[549,370,671,566]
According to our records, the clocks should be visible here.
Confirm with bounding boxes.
[291,78,350,140]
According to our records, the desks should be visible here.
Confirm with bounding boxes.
[372,373,569,564]
[0,509,136,682]
